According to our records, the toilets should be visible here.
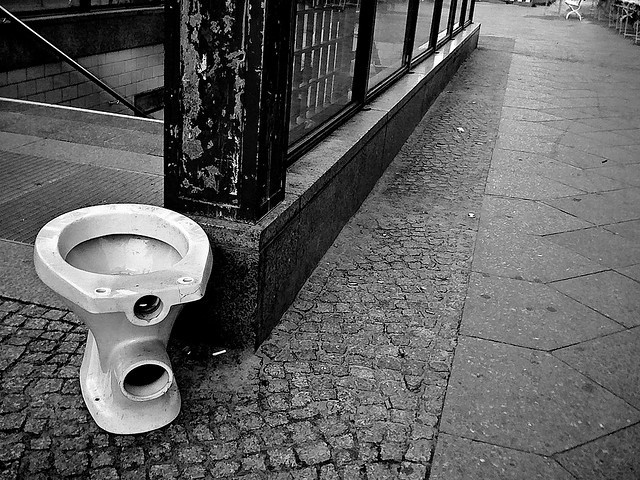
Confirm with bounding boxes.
[34,203,214,436]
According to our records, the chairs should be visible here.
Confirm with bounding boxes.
[565,0,583,21]
